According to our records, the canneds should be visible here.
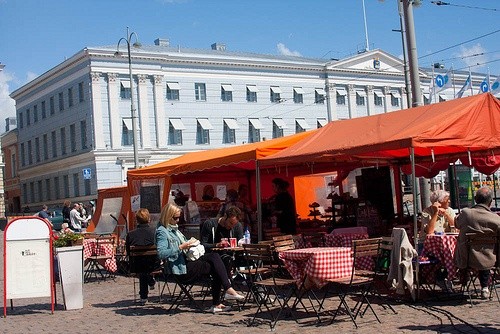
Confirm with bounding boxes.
[230,238,236,248]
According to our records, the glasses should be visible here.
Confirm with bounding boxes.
[172,216,180,221]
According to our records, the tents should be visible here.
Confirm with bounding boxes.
[127,129,324,232]
[86,186,130,236]
[257,94,500,238]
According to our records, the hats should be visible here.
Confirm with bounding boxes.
[89,201,94,206]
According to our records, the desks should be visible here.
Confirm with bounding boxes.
[279,247,356,324]
[84,238,118,272]
[421,234,494,287]
[51,246,84,310]
[317,234,375,271]
[210,244,269,253]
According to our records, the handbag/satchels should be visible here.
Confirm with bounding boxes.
[63,218,70,223]
[183,237,205,261]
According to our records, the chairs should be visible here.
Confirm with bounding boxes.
[82,227,500,330]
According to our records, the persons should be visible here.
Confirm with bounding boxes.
[40,176,500,312]
[452,185,500,300]
[199,205,247,280]
[126,208,157,304]
[154,203,245,312]
[421,190,457,248]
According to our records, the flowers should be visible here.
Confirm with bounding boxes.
[54,231,84,247]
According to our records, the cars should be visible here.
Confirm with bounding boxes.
[49,210,64,230]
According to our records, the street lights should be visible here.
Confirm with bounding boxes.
[114,26,143,171]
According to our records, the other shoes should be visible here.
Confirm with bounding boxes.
[149,278,156,287]
[211,304,231,312]
[140,294,147,299]
[255,292,274,303]
[225,293,244,300]
[481,287,493,298]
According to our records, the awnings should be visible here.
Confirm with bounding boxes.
[391,92,401,99]
[337,90,347,96]
[247,86,260,93]
[167,83,181,90]
[270,87,283,94]
[356,91,367,98]
[315,88,327,95]
[222,85,234,91]
[120,80,139,88]
[294,87,306,95]
[123,117,330,128]
[374,92,385,98]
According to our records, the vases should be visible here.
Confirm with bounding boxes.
[74,238,84,246]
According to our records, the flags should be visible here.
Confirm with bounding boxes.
[456,74,470,100]
[432,67,452,93]
[491,75,500,95]
[478,74,489,95]
